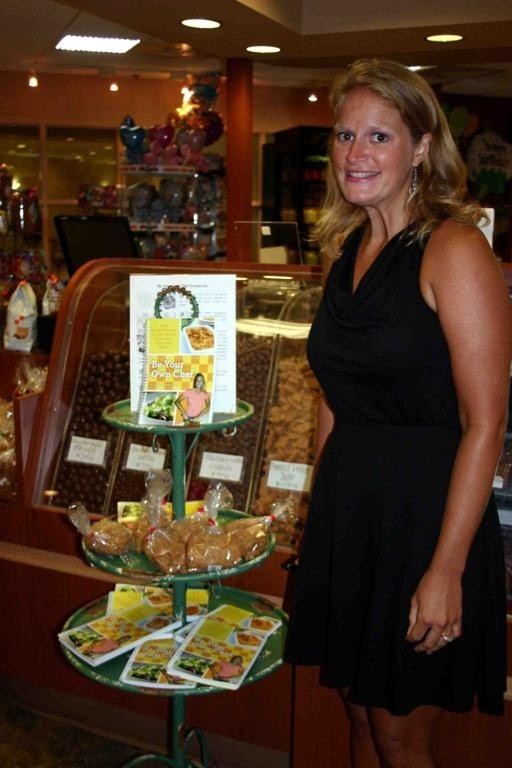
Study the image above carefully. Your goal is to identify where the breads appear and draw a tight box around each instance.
[87,510,270,574]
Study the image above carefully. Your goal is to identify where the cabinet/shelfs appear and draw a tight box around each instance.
[0,259,512,768]
[1,124,264,305]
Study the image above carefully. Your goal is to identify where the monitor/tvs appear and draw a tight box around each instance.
[53,214,140,278]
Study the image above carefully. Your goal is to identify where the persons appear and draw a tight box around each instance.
[83,635,132,657]
[210,656,244,683]
[287,58,512,768]
[175,373,210,426]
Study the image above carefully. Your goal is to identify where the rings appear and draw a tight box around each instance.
[441,635,453,642]
